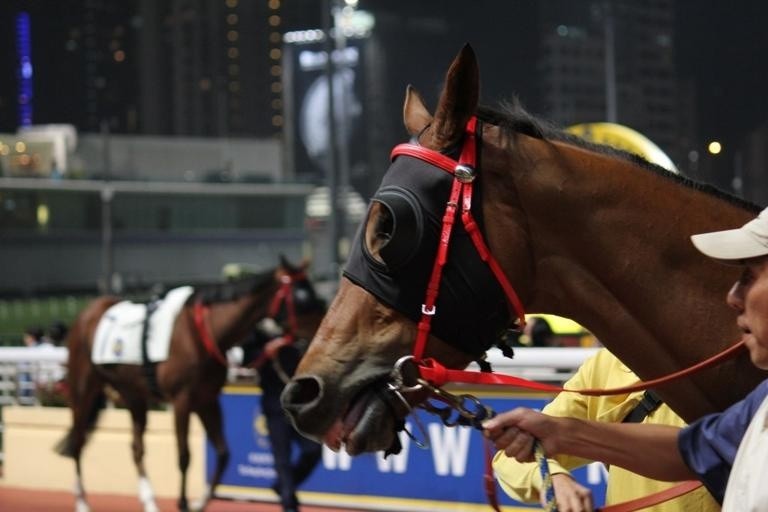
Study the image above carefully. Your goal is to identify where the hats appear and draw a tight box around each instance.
[687,206,768,268]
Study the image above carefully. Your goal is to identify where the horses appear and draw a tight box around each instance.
[279,41,768,457]
[51,253,327,512]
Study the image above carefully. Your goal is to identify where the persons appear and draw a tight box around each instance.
[235,335,325,511]
[485,346,723,512]
[11,320,73,409]
[478,201,767,512]
[521,315,552,347]
